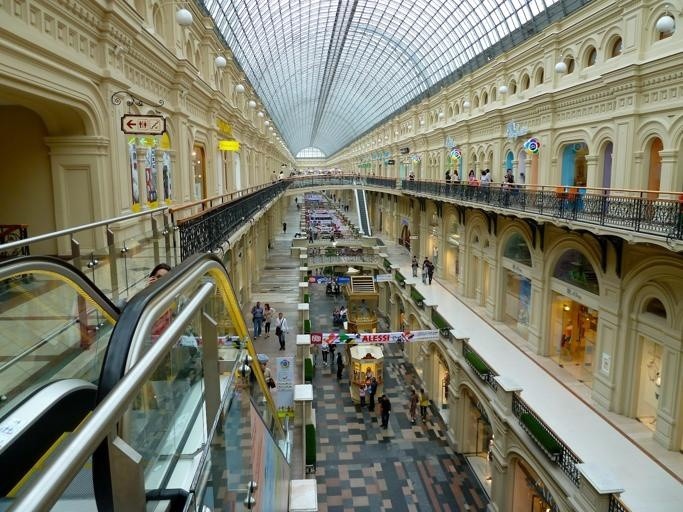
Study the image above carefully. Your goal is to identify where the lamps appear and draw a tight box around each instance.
[554,56,568,76]
[438,110,445,119]
[384,134,389,140]
[655,11,675,35]
[420,119,426,126]
[413,154,421,162]
[499,83,509,95]
[522,137,542,155]
[564,305,570,311]
[450,149,462,161]
[408,124,413,130]
[394,130,399,136]
[153,1,298,165]
[463,99,471,108]
[352,138,382,153]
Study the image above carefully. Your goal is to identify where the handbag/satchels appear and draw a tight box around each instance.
[276,327,283,336]
[270,378,276,388]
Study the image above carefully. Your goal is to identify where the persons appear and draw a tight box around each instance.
[270,171,284,184]
[446,170,491,202]
[412,256,435,285]
[410,389,428,425]
[359,377,391,428]
[333,305,348,328]
[145,264,203,413]
[262,363,271,401]
[337,352,345,378]
[275,313,289,350]
[251,302,276,339]
[326,283,340,295]
[321,344,336,366]
[283,223,286,232]
